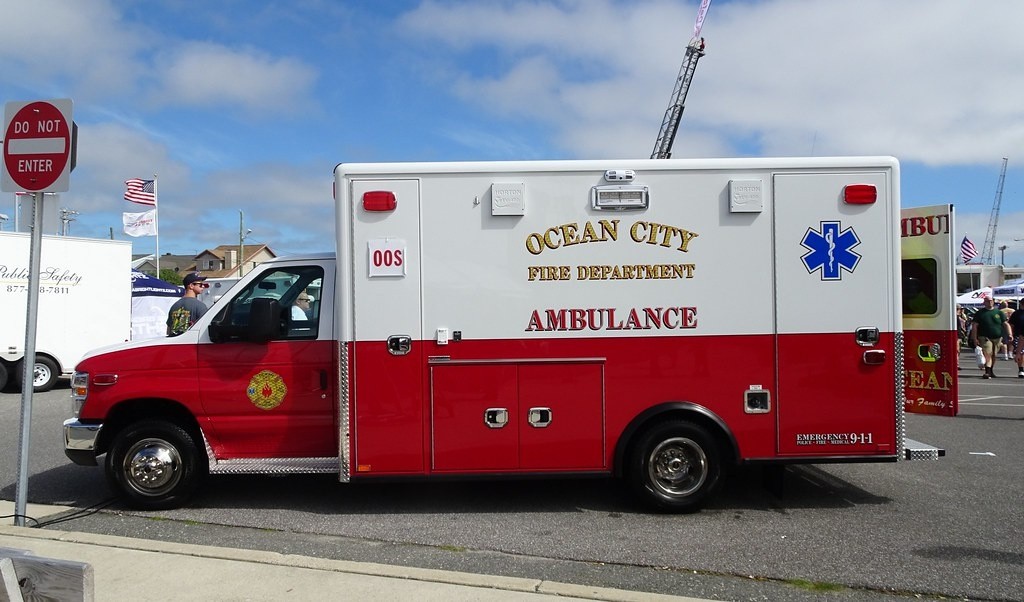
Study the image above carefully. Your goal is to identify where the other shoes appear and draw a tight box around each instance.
[1000,357,1008,360]
[1010,356,1012,358]
[1018,372,1024,379]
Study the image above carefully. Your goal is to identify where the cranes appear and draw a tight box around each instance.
[1013,238,1024,242]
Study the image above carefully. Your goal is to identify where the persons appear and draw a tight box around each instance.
[1015,324,1024,363]
[291,292,310,320]
[166,273,208,337]
[971,295,1014,378]
[957,299,1016,370]
[1008,298,1024,378]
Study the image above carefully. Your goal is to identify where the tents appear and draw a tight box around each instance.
[132,268,184,338]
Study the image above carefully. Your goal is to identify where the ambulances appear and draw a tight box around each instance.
[61,155,960,507]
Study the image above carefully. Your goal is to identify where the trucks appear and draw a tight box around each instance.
[198,276,322,320]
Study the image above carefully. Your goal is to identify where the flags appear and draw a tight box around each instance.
[961,237,979,262]
[123,178,155,205]
[123,209,156,238]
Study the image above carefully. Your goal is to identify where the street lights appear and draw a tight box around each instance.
[239,228,252,278]
[998,246,1006,264]
[980,157,1008,264]
[237,208,245,277]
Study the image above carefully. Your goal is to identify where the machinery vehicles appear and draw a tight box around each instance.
[645,36,706,159]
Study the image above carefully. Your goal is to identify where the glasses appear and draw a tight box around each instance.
[193,283,203,287]
[298,298,311,302]
[984,300,993,303]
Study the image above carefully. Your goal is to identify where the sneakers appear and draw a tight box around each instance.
[983,366,996,380]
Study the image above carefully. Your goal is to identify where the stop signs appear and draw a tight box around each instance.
[1,100,72,192]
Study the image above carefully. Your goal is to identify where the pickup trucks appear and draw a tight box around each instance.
[1,227,134,393]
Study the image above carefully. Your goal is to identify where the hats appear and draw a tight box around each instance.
[182,273,208,287]
